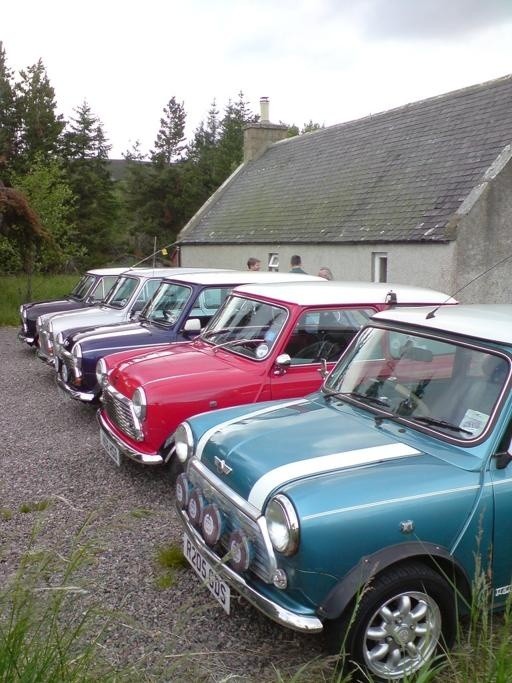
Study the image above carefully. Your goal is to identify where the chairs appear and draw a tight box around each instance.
[454,355,512,444]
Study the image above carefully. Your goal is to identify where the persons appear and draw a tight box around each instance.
[247,258,261,271]
[319,267,333,281]
[288,255,307,274]
